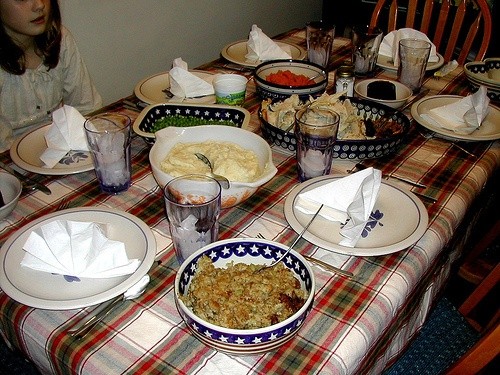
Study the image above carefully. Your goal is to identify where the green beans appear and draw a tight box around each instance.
[151,116,235,132]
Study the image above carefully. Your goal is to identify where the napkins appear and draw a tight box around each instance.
[20,220,142,277]
[293,167,383,248]
[168,58,215,102]
[40,104,99,169]
[378,28,439,62]
[245,24,292,64]
[420,85,490,136]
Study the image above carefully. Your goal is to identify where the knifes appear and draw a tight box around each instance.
[0,161,52,195]
[347,170,438,204]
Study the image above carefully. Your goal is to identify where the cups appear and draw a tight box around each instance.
[294,107,339,180]
[162,174,222,263]
[212,73,249,109]
[306,23,432,93]
[83,114,133,194]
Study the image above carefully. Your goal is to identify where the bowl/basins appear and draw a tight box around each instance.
[174,238,315,356]
[464,57,500,103]
[149,124,278,208]
[354,80,412,107]
[132,102,251,146]
[0,172,22,219]
[252,59,328,104]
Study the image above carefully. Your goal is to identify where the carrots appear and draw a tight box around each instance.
[265,70,317,86]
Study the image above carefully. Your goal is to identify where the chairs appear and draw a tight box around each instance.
[369,0,492,65]
[382,262,500,375]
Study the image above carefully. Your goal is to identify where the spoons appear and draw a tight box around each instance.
[354,163,427,188]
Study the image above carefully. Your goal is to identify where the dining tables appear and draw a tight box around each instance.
[0,26,500,375]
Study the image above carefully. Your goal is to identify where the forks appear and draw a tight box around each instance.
[417,127,478,160]
[255,234,353,279]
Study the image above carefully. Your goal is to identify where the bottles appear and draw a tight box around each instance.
[334,60,356,98]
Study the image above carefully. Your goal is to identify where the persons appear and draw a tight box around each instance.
[0,0,104,157]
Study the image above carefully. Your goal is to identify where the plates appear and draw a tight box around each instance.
[222,38,306,67]
[135,69,216,104]
[10,115,125,175]
[376,51,444,72]
[284,175,428,258]
[0,205,156,310]
[411,94,500,142]
